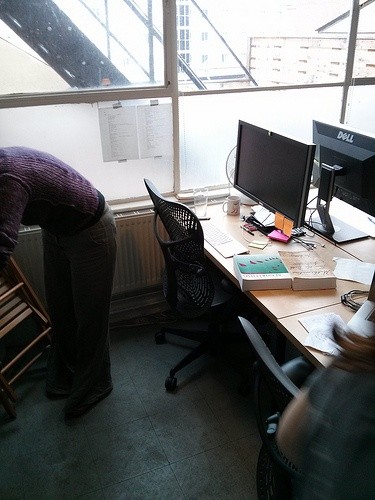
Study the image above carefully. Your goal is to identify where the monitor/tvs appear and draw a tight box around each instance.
[234,119,316,237]
[304,119,375,243]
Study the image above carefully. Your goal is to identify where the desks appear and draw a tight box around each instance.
[166,185,374,372]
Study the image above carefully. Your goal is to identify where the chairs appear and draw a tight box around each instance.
[0,255,51,417]
[237,316,301,500]
[143,179,247,392]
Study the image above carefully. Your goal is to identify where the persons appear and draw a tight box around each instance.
[276,325,375,500]
[0,146,118,420]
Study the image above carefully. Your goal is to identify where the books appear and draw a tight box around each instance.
[278,250,337,290]
[233,252,292,292]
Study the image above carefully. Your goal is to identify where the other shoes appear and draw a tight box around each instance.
[45,388,68,400]
[62,387,112,418]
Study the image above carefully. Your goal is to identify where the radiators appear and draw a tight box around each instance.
[12,207,169,311]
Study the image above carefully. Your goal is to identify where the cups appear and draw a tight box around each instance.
[222,195,240,215]
[193,187,209,217]
